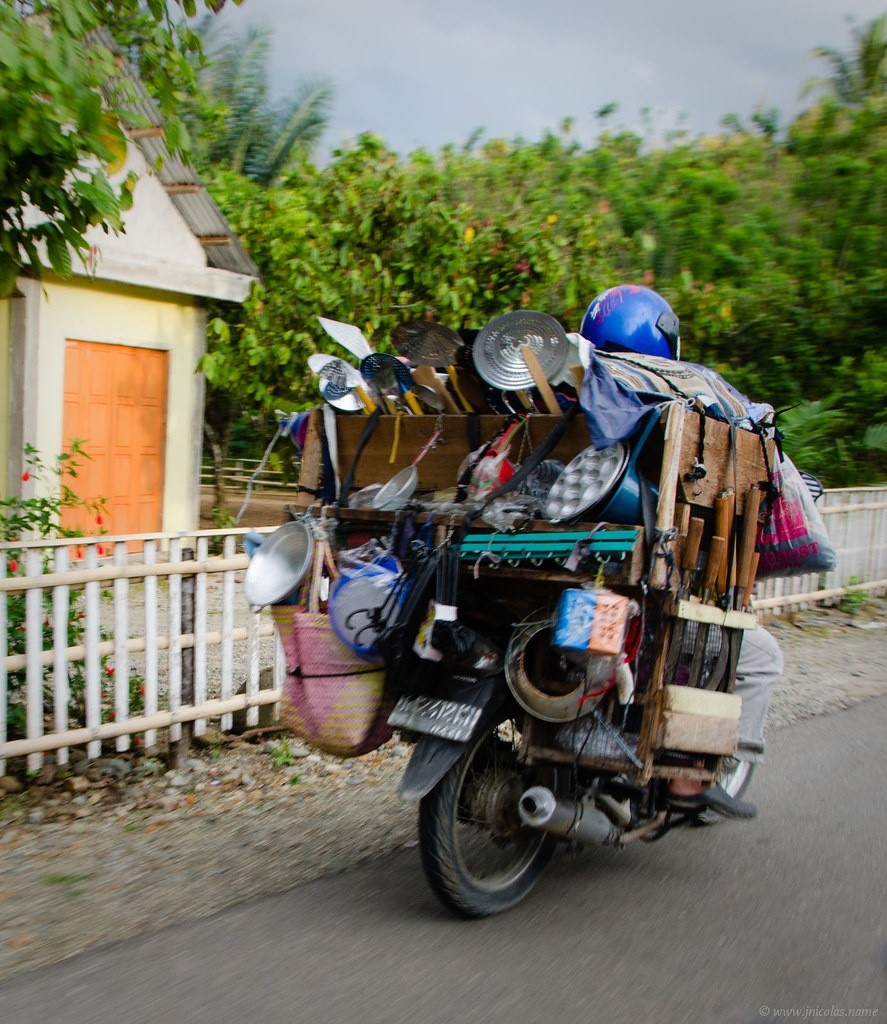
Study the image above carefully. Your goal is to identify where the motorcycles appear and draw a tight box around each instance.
[387,585,760,921]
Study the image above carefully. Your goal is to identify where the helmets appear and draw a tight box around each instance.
[579,284,679,361]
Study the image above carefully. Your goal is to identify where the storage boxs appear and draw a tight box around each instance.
[312,402,774,522]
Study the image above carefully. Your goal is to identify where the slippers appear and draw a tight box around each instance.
[656,779,757,820]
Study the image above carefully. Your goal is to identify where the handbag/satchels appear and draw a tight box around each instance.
[754,441,836,581]
[271,538,398,759]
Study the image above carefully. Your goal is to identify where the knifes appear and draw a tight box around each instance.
[665,484,762,694]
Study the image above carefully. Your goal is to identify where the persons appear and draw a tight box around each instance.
[578,282,785,822]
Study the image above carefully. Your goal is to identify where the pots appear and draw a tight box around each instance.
[244,520,312,614]
